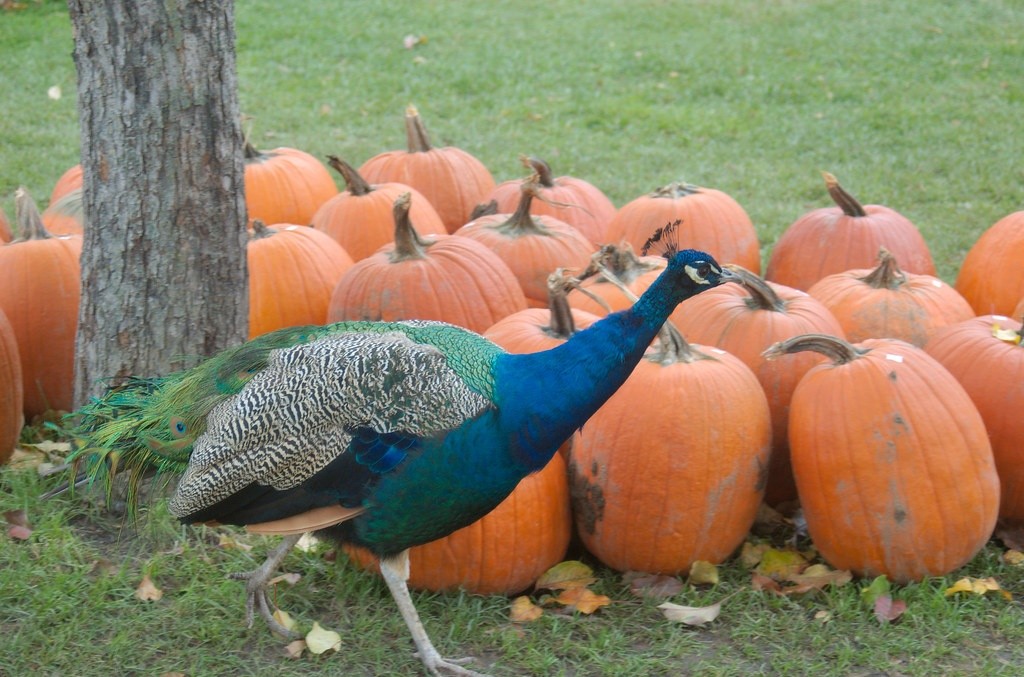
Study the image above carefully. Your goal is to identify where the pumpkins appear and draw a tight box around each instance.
[242,105,1024,594]
[0,163,84,467]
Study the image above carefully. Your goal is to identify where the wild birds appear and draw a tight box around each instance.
[65,221,752,677]
